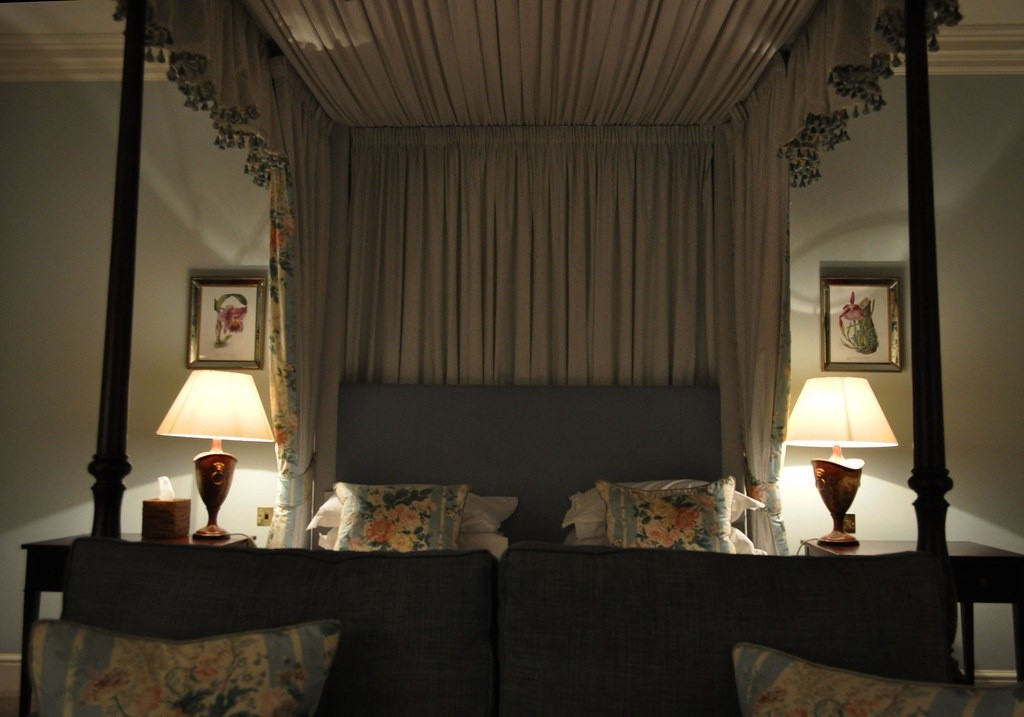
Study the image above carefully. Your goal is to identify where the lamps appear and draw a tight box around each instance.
[784,377,900,548]
[156,370,276,540]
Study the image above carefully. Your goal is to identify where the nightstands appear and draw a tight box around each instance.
[801,538,1024,687]
[19,533,256,716]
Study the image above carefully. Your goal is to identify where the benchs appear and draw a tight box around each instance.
[63,537,954,717]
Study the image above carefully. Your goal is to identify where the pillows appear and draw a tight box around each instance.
[733,641,1024,717]
[26,614,347,717]
[561,475,769,556]
[304,480,518,561]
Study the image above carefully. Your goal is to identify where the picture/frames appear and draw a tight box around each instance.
[818,277,902,373]
[185,276,267,369]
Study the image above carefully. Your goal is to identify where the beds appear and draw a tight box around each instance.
[87,0,962,554]
